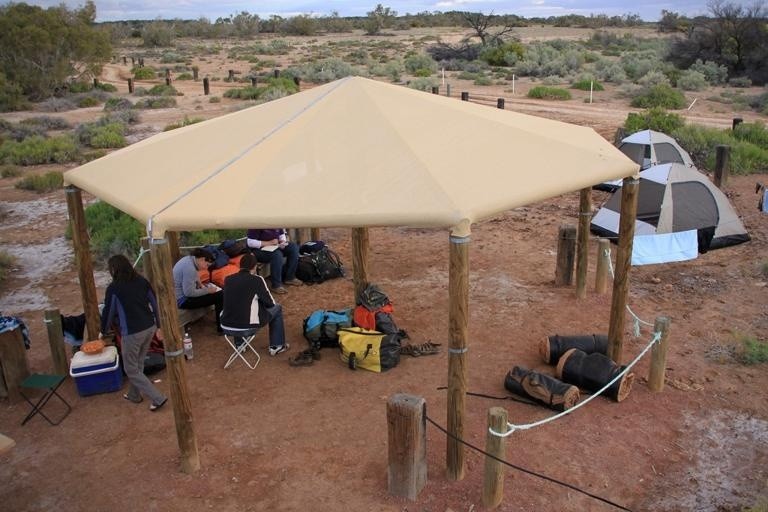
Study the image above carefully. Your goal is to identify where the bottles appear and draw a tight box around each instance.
[183,333,194,360]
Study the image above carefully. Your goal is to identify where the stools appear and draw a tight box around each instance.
[17,370,73,427]
[219,319,263,371]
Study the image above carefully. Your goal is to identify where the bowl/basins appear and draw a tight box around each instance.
[80,339,105,354]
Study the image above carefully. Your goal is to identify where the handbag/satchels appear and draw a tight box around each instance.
[302,308,351,349]
[336,327,402,373]
[352,285,405,337]
[296,240,344,282]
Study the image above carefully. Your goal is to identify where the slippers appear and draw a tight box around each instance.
[124,392,143,402]
[149,398,168,411]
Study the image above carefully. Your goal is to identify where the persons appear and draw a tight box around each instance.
[173,249,224,335]
[220,254,290,356]
[97,255,167,411]
[164,68,174,85]
[247,226,302,294]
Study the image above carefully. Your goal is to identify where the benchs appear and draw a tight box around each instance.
[177,253,289,343]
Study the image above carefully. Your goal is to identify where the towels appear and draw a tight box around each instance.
[630,228,699,266]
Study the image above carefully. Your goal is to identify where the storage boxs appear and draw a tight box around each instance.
[69,348,125,395]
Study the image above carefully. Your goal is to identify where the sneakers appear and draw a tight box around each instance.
[400,339,442,356]
[289,348,321,366]
[285,278,303,286]
[269,342,290,356]
[271,286,288,294]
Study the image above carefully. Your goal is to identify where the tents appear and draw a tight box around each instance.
[591,129,697,193]
[591,161,753,252]
